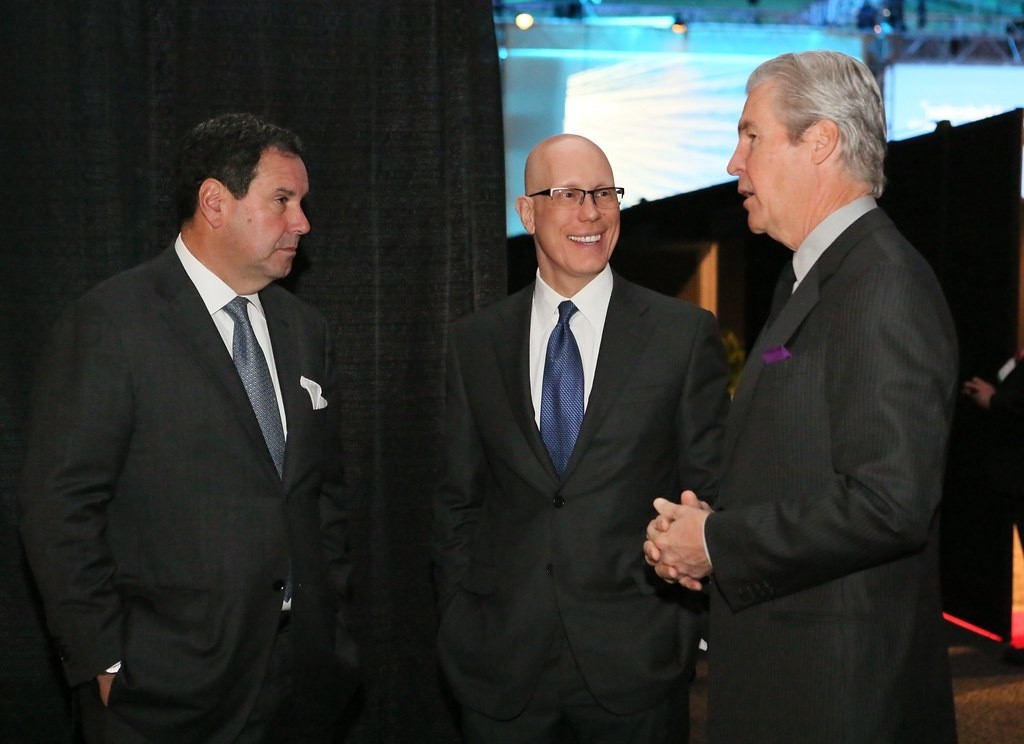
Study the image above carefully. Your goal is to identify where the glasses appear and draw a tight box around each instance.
[527,187,625,209]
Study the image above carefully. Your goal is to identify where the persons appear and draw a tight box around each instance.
[12,112,345,744]
[960,340,1024,554]
[428,133,732,744]
[642,51,960,744]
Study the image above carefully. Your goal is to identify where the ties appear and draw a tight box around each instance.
[540,301,584,479]
[223,294,291,603]
[766,259,795,333]
